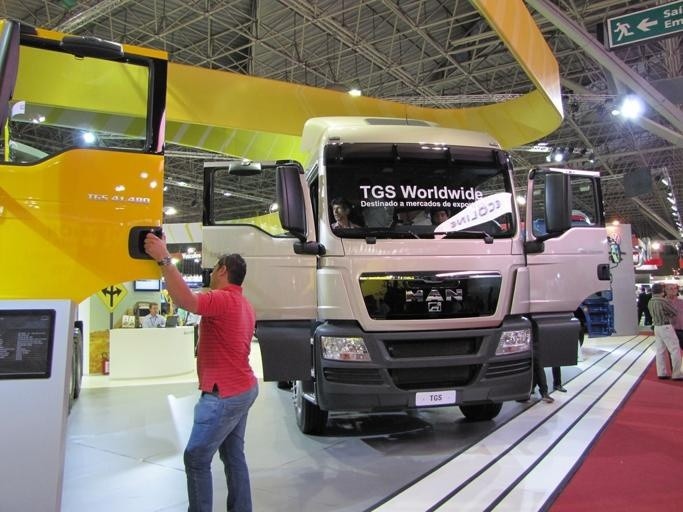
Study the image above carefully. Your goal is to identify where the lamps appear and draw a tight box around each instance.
[660,167,682,243]
[545,147,596,165]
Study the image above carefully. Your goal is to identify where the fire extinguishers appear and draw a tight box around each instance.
[102,358,110,375]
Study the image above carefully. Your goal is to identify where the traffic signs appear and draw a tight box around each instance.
[607,1,683,50]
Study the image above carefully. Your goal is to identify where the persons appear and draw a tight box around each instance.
[141,302,167,328]
[331,197,361,230]
[141,232,259,511]
[635,283,682,381]
[184,310,202,357]
[529,364,554,402]
[551,366,568,393]
[431,209,450,233]
[611,20,636,42]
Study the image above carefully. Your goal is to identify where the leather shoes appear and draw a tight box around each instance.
[554,384,567,392]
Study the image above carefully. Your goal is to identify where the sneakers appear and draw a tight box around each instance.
[542,394,553,402]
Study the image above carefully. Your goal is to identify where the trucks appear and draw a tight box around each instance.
[199,114,616,442]
[521,214,617,352]
[0,13,171,423]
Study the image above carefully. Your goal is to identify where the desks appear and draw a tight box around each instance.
[108,324,196,380]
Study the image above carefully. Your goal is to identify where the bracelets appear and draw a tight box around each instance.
[155,255,173,267]
